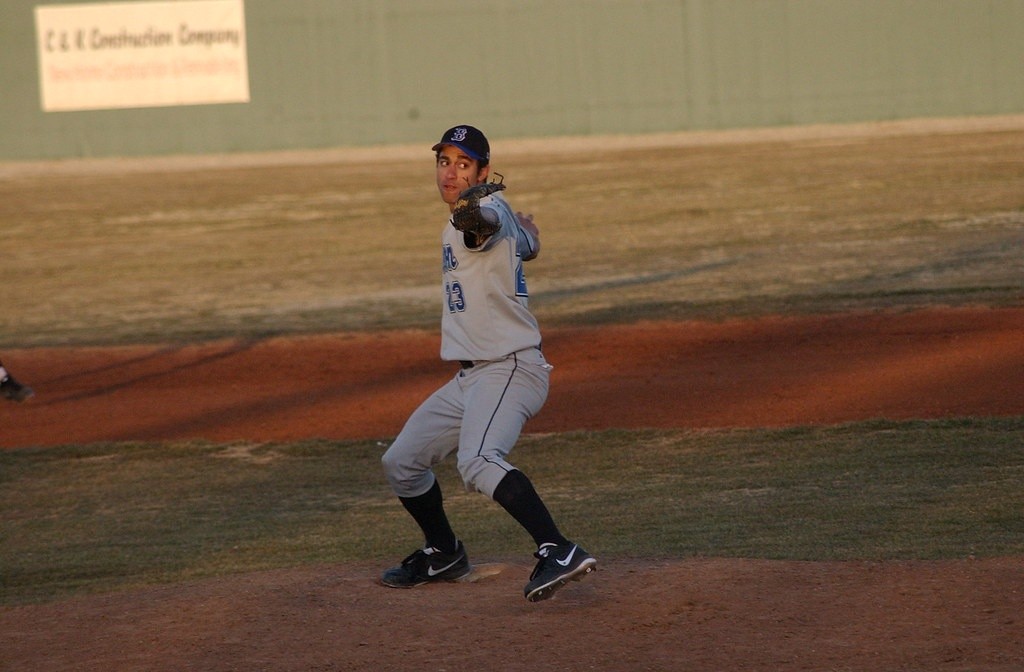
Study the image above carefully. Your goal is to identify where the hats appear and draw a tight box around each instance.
[432,125,490,165]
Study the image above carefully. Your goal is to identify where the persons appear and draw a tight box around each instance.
[380,124,597,602]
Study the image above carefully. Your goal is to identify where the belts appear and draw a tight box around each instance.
[460,343,541,370]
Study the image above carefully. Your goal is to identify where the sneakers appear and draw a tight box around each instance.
[380,540,470,589]
[0,375,35,404]
[525,542,597,602]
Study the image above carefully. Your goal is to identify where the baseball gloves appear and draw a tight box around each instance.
[449,171,506,246]
